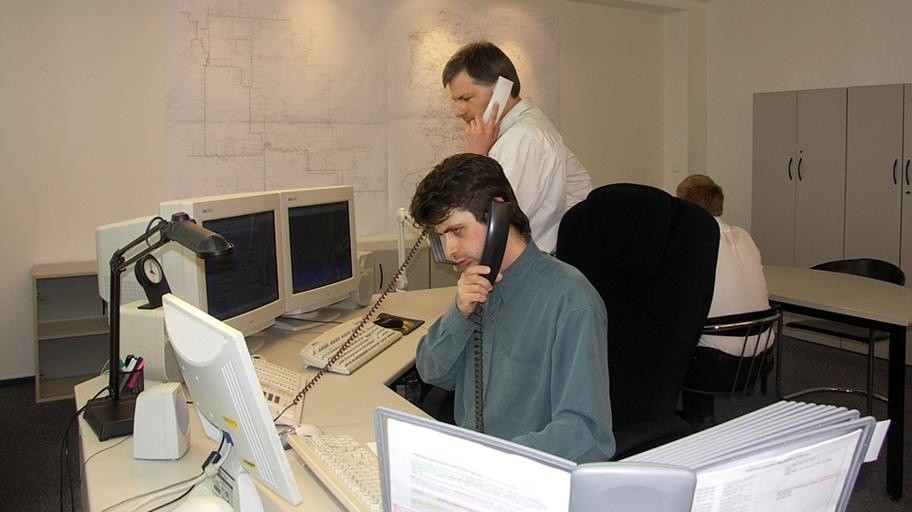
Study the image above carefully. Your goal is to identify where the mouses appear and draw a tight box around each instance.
[277,422,324,450]
[375,314,405,328]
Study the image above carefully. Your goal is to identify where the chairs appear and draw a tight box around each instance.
[680,307,781,417]
[773,253,907,426]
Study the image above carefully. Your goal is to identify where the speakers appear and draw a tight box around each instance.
[130,382,193,463]
[119,297,187,382]
[350,251,375,307]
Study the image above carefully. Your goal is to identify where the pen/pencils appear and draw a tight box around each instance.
[117,355,145,396]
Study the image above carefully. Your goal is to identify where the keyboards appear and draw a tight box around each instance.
[287,431,382,512]
[300,315,403,375]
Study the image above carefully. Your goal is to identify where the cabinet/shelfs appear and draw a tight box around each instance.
[839,84,911,278]
[28,260,113,405]
[750,82,848,267]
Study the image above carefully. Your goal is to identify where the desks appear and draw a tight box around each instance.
[74,258,911,512]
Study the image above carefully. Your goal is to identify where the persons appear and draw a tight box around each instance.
[441,41,594,259]
[411,152,619,465]
[677,175,774,427]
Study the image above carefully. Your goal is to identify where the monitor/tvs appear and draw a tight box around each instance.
[160,292,304,512]
[281,185,361,331]
[94,191,286,354]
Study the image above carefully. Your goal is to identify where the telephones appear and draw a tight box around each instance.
[479,199,513,286]
[482,75,514,127]
